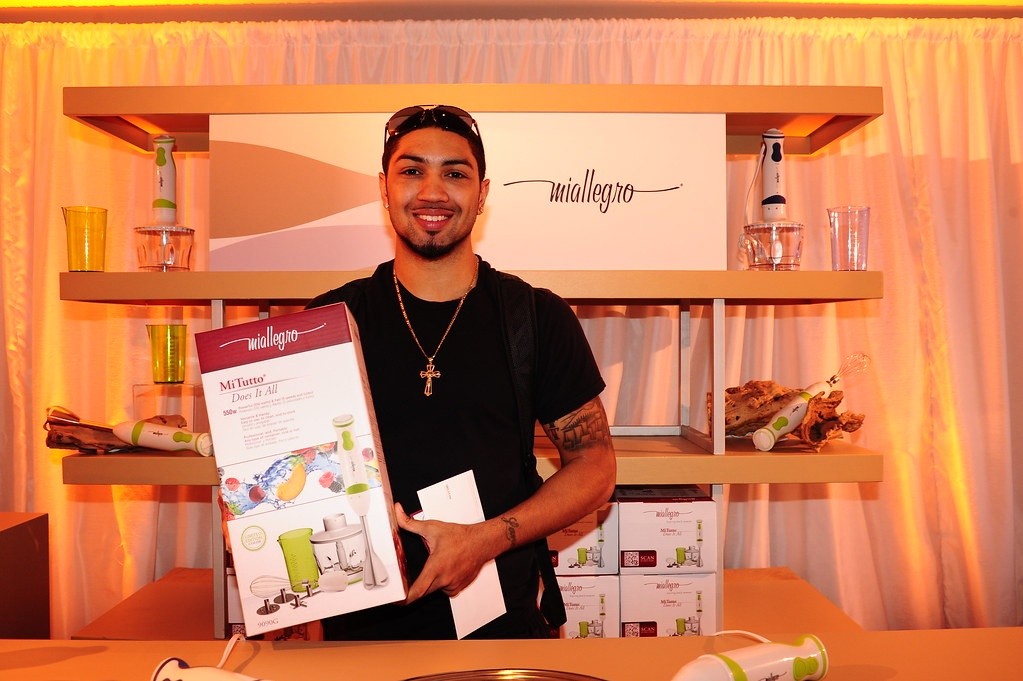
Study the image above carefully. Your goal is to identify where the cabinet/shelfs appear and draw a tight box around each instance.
[57,84,885,641]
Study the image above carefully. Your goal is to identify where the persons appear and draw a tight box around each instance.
[217,104,617,640]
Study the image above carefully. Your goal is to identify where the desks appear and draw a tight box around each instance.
[0,626,1023,681]
[0,510,51,643]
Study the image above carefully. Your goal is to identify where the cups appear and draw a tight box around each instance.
[676,618,685,634]
[676,547,685,563]
[826,205,871,271]
[579,621,589,638]
[145,324,188,383]
[577,548,587,564]
[277,528,320,593]
[62,206,108,272]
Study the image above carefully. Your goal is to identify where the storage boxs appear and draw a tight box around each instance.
[194,302,407,642]
[534,486,720,636]
[207,114,728,271]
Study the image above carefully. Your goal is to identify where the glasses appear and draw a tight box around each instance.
[384,105,482,150]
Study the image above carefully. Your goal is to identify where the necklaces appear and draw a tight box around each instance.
[393,254,479,395]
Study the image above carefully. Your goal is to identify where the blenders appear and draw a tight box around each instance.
[133,134,196,272]
[743,129,805,271]
